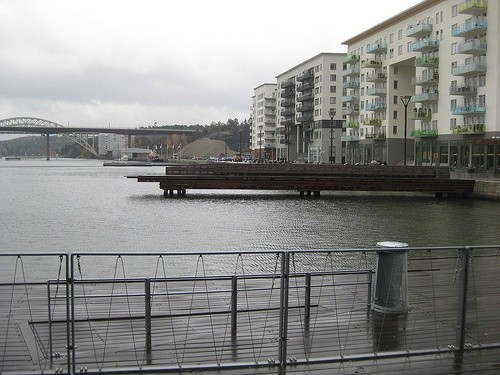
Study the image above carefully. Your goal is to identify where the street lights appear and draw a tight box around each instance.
[286,119,292,162]
[238,129,243,158]
[258,125,263,158]
[400,94,412,166]
[328,108,337,165]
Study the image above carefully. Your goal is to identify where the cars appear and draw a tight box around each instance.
[169,153,389,168]
